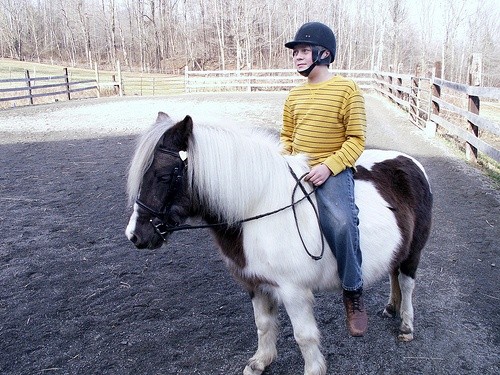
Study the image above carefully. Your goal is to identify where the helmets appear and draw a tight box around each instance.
[284,22,336,63]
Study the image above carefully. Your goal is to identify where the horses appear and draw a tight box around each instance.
[123,112,433,375]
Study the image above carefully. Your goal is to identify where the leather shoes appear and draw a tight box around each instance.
[343,289,368,337]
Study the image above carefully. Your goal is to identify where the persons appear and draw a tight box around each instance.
[281,23,369,336]
